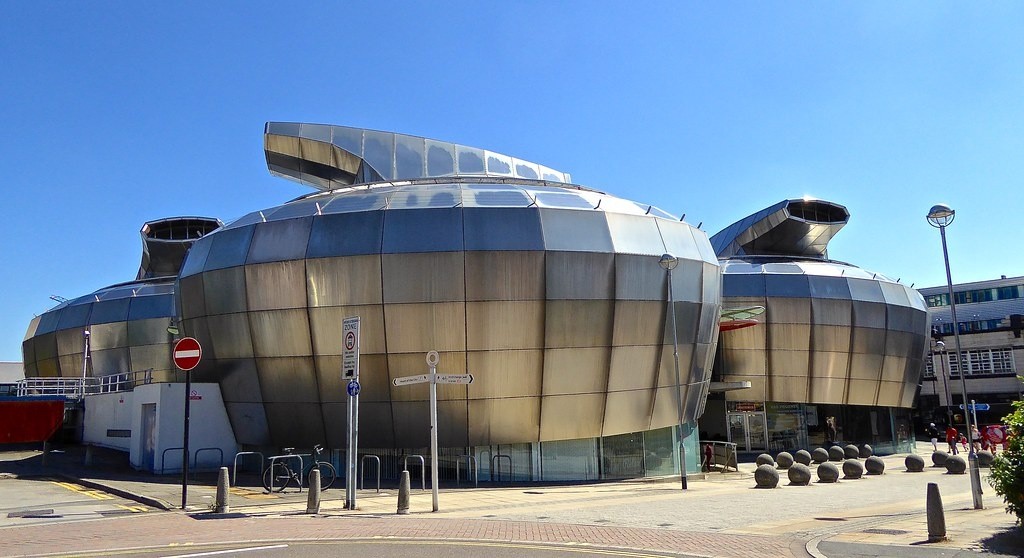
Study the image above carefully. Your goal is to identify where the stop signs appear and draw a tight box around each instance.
[172,336,203,370]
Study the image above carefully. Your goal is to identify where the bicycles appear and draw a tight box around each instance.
[262,442,337,493]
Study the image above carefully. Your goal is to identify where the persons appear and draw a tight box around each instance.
[704,444,712,472]
[958,433,968,452]
[929,423,939,451]
[971,425,980,453]
[945,424,959,454]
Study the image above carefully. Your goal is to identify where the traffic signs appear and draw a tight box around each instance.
[392,374,430,387]
[435,373,475,385]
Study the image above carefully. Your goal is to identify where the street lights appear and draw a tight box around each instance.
[935,340,955,429]
[656,254,690,492]
[925,202,985,511]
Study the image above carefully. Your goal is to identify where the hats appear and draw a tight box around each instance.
[930,423,935,427]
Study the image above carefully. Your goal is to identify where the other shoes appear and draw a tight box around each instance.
[947,451,951,453]
